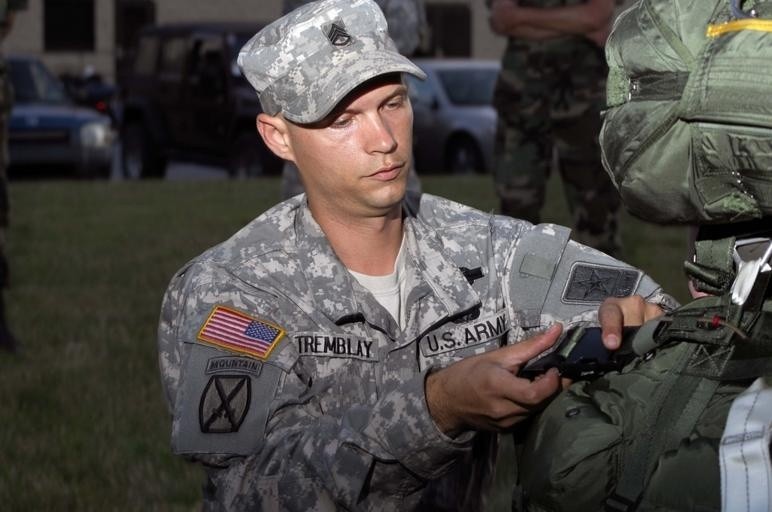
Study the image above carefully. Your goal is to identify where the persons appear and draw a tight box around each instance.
[0,0,30,354]
[483,0,623,256]
[84,68,120,130]
[158,1,683,512]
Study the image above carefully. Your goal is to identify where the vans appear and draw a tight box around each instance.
[118,25,287,180]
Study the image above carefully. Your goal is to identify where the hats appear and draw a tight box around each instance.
[236,0,426,124]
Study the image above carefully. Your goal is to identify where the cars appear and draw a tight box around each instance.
[0,59,122,180]
[405,59,500,174]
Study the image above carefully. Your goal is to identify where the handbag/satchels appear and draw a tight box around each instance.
[458,296,771,511]
[598,0,772,223]
